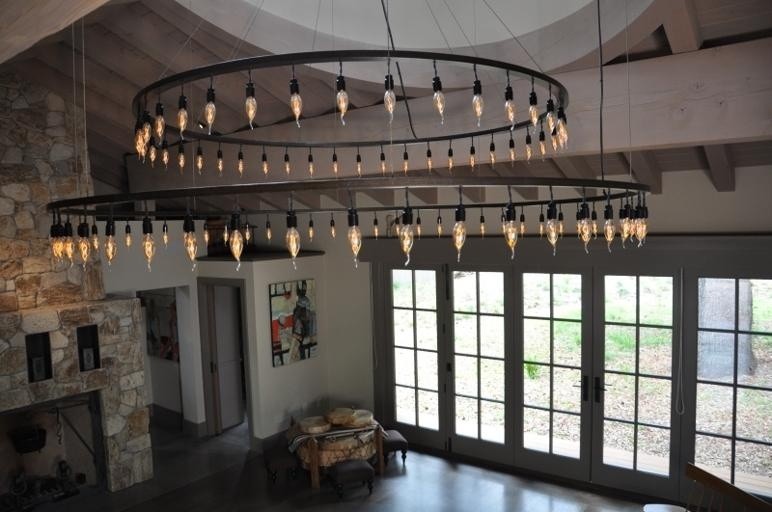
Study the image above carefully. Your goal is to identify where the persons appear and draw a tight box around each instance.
[292,280,311,361]
[282,289,292,316]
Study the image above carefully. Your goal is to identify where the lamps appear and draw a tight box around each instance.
[47,0,651,271]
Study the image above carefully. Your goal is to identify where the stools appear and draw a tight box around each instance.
[327,458,376,499]
[263,442,309,501]
[382,430,408,466]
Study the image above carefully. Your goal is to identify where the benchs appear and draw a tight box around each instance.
[642,460,772,512]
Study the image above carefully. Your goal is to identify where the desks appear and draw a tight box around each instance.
[279,419,388,489]
